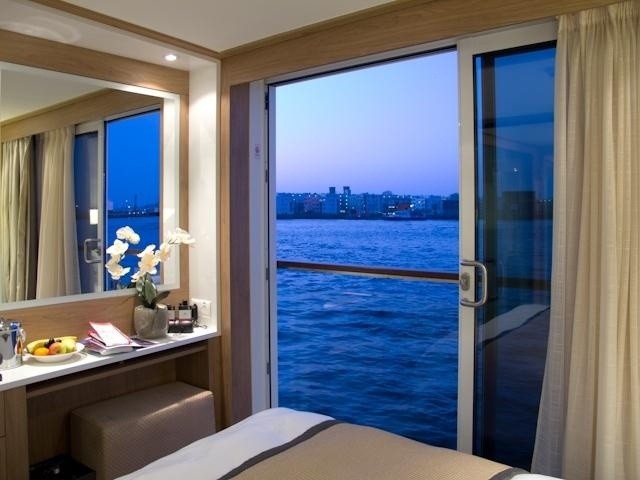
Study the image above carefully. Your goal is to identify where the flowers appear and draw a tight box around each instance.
[130,226,196,310]
[103,225,140,290]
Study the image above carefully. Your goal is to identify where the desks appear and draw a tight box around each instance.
[0,325,224,480]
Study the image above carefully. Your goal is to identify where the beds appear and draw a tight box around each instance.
[110,404,565,480]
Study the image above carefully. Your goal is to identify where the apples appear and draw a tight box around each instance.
[49,343,67,355]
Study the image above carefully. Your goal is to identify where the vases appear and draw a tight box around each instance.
[134,304,169,340]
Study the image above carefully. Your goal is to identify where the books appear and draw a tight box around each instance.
[85,321,157,357]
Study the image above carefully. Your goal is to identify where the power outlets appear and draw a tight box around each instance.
[191,299,211,320]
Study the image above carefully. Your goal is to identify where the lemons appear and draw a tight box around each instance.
[34,347,49,356]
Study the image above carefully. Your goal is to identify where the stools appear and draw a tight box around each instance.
[67,380,218,480]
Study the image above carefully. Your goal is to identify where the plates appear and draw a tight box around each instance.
[24,343,85,363]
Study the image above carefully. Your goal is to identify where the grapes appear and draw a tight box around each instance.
[44,338,61,348]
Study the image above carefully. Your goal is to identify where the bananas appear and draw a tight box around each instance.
[26,335,78,353]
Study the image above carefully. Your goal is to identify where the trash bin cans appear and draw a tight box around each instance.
[29,453,97,480]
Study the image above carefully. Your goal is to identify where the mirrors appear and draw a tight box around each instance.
[0,29,190,345]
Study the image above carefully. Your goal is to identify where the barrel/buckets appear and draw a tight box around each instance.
[0,317,26,371]
